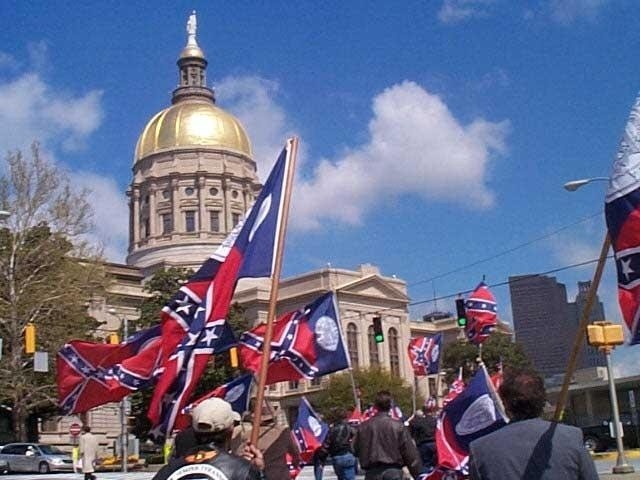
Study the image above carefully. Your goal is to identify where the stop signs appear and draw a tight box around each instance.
[69,423,81,437]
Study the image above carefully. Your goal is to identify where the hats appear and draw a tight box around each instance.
[192,396,241,434]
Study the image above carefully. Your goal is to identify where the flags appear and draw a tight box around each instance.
[407,331,441,376]
[56,318,238,415]
[285,396,328,479]
[423,369,509,478]
[145,139,292,444]
[236,292,349,386]
[464,281,498,345]
[604,90,640,346]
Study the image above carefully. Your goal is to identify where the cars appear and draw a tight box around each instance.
[0,442,74,474]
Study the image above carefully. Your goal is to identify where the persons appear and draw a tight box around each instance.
[468,365,600,478]
[311,390,437,480]
[151,396,258,478]
[77,426,99,480]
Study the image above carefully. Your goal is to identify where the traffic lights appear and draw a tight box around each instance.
[372,316,385,344]
[454,298,468,326]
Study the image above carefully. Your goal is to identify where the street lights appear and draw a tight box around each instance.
[564,177,610,192]
